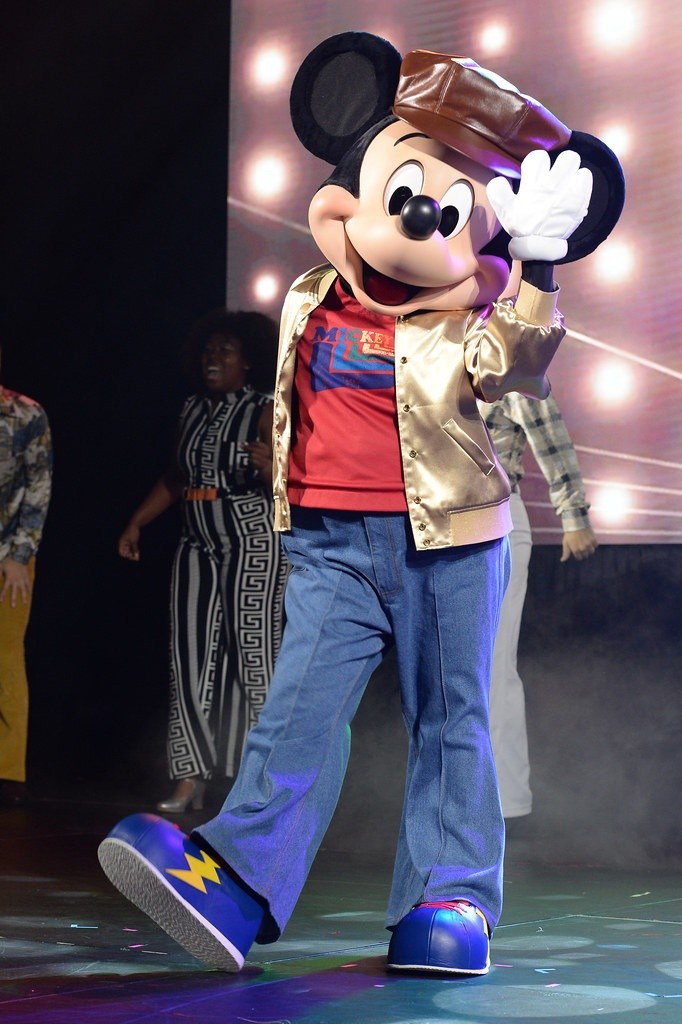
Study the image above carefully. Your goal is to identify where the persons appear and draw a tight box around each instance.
[472,383,599,820]
[0,384,53,809]
[122,310,293,815]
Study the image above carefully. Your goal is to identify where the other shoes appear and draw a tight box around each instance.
[5,781,29,805]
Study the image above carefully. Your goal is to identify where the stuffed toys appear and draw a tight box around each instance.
[97,32,625,974]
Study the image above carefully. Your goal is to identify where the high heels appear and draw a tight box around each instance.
[157,777,204,812]
